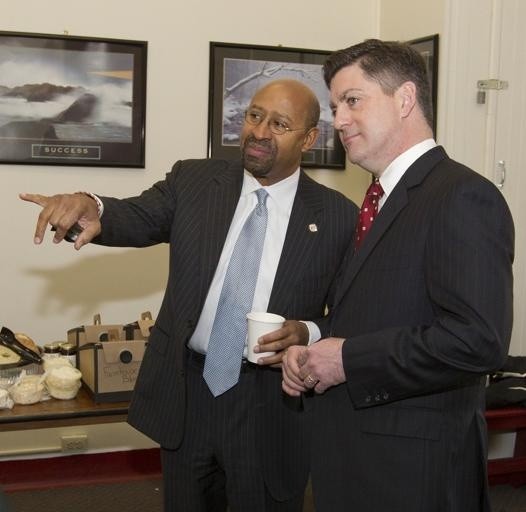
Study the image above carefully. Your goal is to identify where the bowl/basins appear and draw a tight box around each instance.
[9,368,82,405]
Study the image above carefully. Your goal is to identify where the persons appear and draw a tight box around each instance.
[18,80,363,511]
[280,37,516,512]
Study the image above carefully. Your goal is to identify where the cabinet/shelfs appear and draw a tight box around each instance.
[0,361,524,494]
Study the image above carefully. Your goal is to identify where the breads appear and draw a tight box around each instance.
[15,333,40,355]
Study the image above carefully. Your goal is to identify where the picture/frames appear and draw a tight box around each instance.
[1,28,145,170]
[205,33,440,170]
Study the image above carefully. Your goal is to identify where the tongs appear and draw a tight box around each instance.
[0,326,45,364]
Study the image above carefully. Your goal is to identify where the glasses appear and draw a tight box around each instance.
[245,110,308,135]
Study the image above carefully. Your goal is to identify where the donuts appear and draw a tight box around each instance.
[0,344,21,364]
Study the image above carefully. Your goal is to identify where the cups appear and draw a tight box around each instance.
[245,313,285,364]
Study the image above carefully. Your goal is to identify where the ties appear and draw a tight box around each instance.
[203,188,268,398]
[354,184,384,255]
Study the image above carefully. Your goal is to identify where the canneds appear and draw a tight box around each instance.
[44,342,77,368]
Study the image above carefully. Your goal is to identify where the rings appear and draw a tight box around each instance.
[306,374,316,385]
